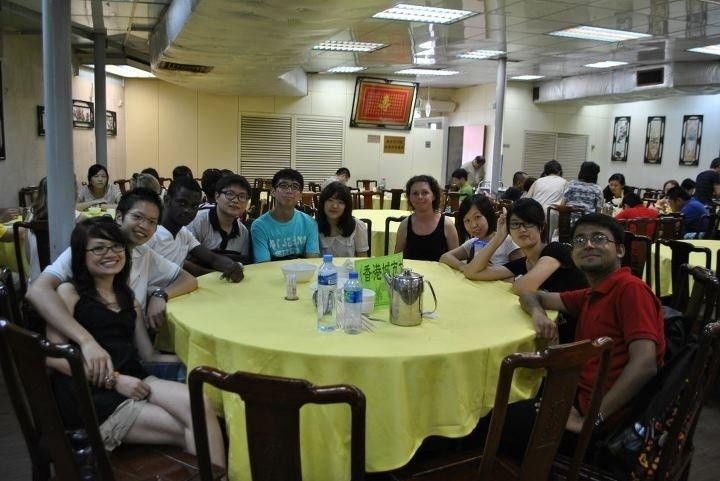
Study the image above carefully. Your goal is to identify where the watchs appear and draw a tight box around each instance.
[595,410,605,429]
[102,371,121,390]
[151,288,169,303]
[233,262,244,271]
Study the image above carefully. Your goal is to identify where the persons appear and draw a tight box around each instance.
[445,169,472,216]
[465,198,587,342]
[652,180,681,213]
[180,175,249,276]
[561,162,605,227]
[528,161,569,229]
[666,187,712,240]
[173,165,193,178]
[438,194,527,273]
[27,176,92,289]
[613,193,658,258]
[74,164,123,210]
[24,187,200,399]
[394,174,459,261]
[601,173,634,204]
[520,177,537,199]
[682,179,696,197]
[251,169,319,263]
[321,167,351,191]
[478,212,665,457]
[316,181,371,256]
[461,155,486,194]
[142,176,244,283]
[131,174,161,195]
[0,207,30,242]
[202,169,225,204]
[42,217,228,480]
[694,157,719,204]
[502,172,530,201]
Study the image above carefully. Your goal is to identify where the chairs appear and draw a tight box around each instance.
[671,262,719,345]
[353,190,383,211]
[253,178,273,191]
[114,180,131,195]
[654,216,684,238]
[308,182,322,194]
[0,322,226,481]
[189,366,367,481]
[618,216,659,239]
[654,236,712,313]
[241,188,271,230]
[414,338,612,479]
[384,216,409,258]
[442,192,469,219]
[381,189,408,210]
[621,230,652,288]
[13,220,51,296]
[559,319,719,481]
[159,177,172,192]
[546,204,587,246]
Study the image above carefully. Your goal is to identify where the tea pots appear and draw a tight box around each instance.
[382,267,438,328]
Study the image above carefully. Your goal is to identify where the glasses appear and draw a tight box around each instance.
[85,242,125,255]
[571,234,621,245]
[509,220,537,229]
[221,191,249,202]
[275,182,300,190]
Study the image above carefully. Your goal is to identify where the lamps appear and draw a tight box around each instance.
[425,86,431,117]
[81,58,157,77]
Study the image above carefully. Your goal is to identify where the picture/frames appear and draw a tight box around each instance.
[350,76,419,131]
[644,116,666,163]
[105,110,117,135]
[38,105,45,135]
[72,99,94,128]
[611,116,631,161]
[678,114,703,166]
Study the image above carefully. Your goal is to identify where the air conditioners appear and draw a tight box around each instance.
[421,100,456,112]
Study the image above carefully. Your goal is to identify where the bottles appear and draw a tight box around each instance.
[604,202,612,217]
[344,272,362,335]
[317,255,338,332]
[381,178,386,187]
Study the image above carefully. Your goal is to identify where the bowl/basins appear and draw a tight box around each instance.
[363,301,374,312]
[362,289,376,301]
[280,263,316,283]
